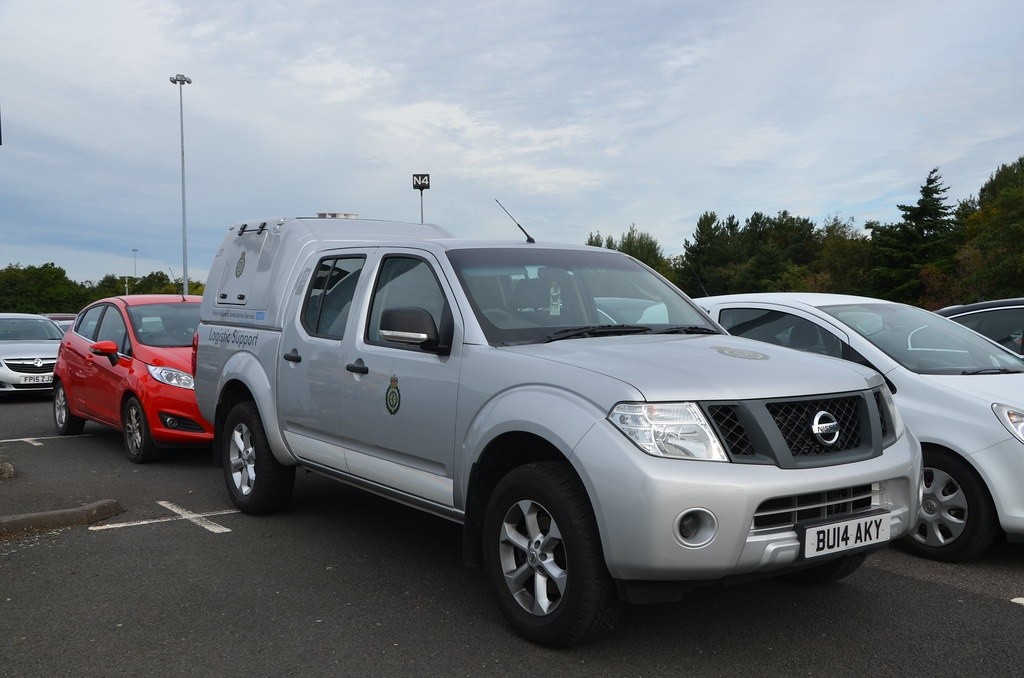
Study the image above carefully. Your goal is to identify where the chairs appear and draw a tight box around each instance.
[746,316,784,346]
[109,309,199,354]
[462,270,485,311]
[507,278,558,327]
[788,321,821,355]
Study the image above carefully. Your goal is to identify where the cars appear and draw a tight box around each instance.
[0,313,65,394]
[691,291,1024,566]
[51,294,220,462]
[930,298,1024,358]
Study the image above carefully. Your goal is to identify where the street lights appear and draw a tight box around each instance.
[170,74,192,295]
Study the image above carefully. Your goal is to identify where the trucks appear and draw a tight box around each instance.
[190,211,924,651]
